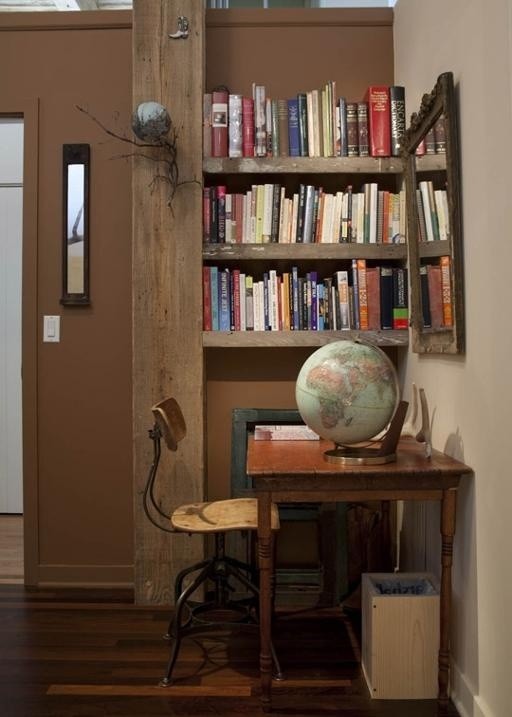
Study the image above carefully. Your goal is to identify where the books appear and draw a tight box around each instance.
[253,423,320,441]
[203,183,407,244]
[409,116,455,329]
[202,81,407,158]
[202,259,407,331]
[271,565,326,613]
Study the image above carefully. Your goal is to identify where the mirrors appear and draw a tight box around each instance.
[60,143,91,306]
[397,70,467,355]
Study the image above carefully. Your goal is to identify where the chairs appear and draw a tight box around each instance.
[143,394,289,684]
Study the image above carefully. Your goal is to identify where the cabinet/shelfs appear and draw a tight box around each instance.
[202,156,409,348]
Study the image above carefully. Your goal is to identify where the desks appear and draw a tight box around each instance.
[245,425,473,707]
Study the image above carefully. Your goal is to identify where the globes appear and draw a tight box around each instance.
[294,334,409,465]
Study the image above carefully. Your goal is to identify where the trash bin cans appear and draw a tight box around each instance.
[361,572,440,699]
